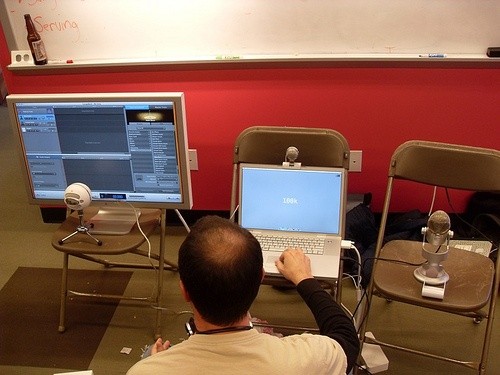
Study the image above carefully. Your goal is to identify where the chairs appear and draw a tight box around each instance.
[224,122,350,346]
[50,201,181,344]
[352,138,500,373]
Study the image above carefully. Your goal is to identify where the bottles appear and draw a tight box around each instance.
[23,14,48,65]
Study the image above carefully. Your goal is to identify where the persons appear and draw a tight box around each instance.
[124,215,362,375]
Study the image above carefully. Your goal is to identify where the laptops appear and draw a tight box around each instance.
[239,163,345,281]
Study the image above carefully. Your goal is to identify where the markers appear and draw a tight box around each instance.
[47,60,73,65]
[418,54,445,58]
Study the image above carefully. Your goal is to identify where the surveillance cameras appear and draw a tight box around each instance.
[57,183,102,246]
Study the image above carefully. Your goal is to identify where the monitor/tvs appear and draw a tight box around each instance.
[5,91,193,210]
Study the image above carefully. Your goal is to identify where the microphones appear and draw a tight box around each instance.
[421,210,454,246]
[285,146,299,162]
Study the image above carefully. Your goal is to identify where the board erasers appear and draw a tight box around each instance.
[487,47,500,57]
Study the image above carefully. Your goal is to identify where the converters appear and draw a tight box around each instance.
[357,331,390,374]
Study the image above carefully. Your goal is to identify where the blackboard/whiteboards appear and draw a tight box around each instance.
[0,1,500,67]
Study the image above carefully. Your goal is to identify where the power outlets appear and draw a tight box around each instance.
[347,149,362,172]
[186,148,199,171]
